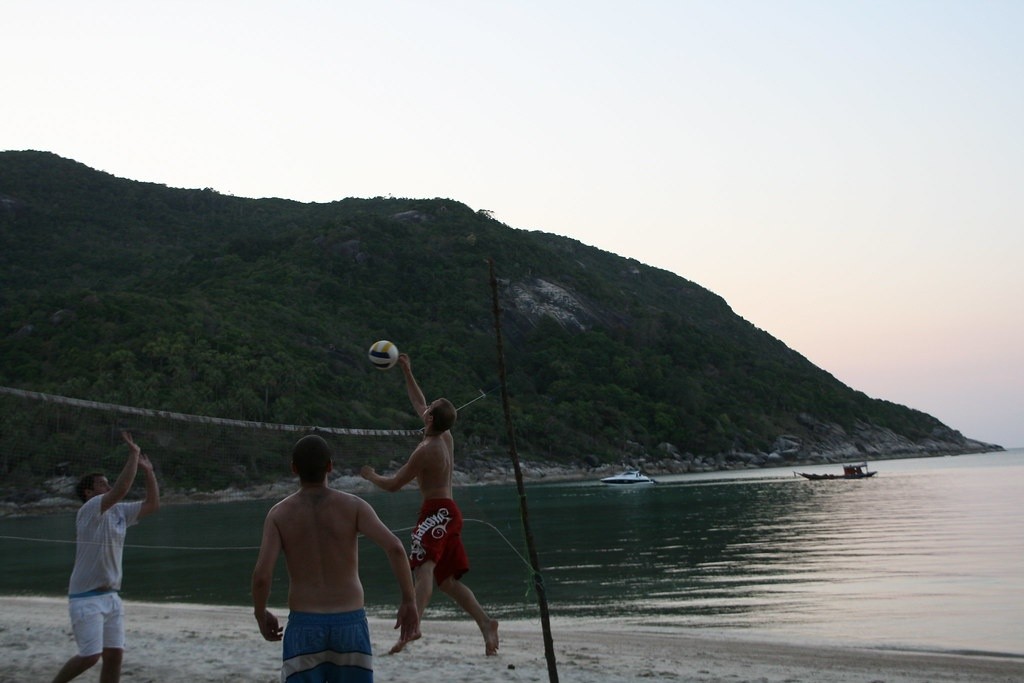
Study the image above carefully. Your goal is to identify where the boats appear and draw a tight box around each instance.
[794,461,878,481]
[600,470,657,485]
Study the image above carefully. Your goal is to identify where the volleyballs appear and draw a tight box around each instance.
[369,339,398,370]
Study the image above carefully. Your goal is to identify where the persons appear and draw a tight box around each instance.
[360,353,500,655]
[249,435,421,682]
[51,431,161,683]
